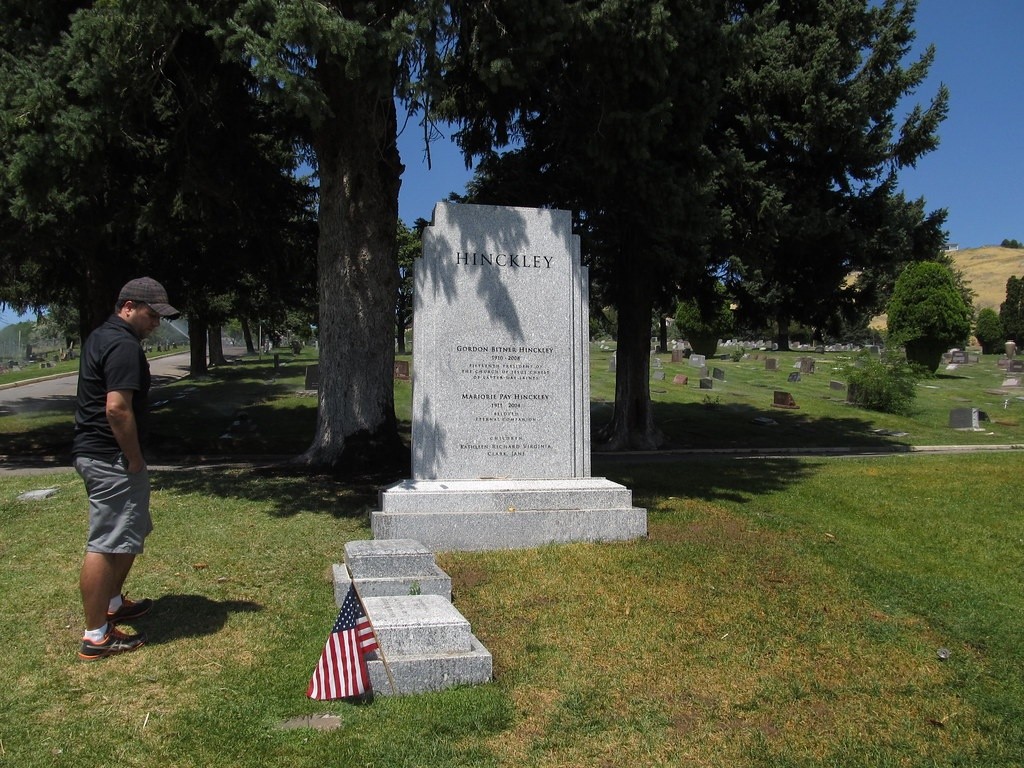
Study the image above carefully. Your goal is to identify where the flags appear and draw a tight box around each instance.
[308,584,379,701]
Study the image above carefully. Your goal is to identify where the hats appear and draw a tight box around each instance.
[118,276,181,320]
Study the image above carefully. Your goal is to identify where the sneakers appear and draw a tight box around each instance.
[107,591,154,623]
[78,623,148,659]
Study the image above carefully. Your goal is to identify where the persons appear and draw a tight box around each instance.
[74,277,178,659]
